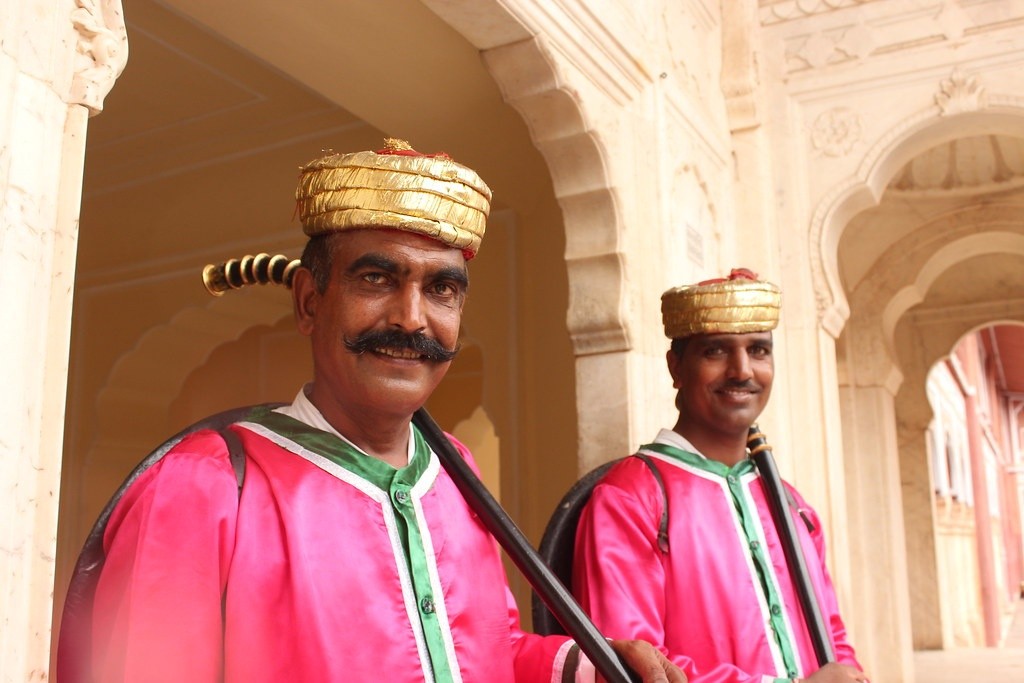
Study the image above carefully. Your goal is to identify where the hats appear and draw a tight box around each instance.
[661,268,781,338]
[298,138,493,260]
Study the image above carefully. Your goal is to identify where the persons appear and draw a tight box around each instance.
[61,137,696,682]
[574,270,871,683]
